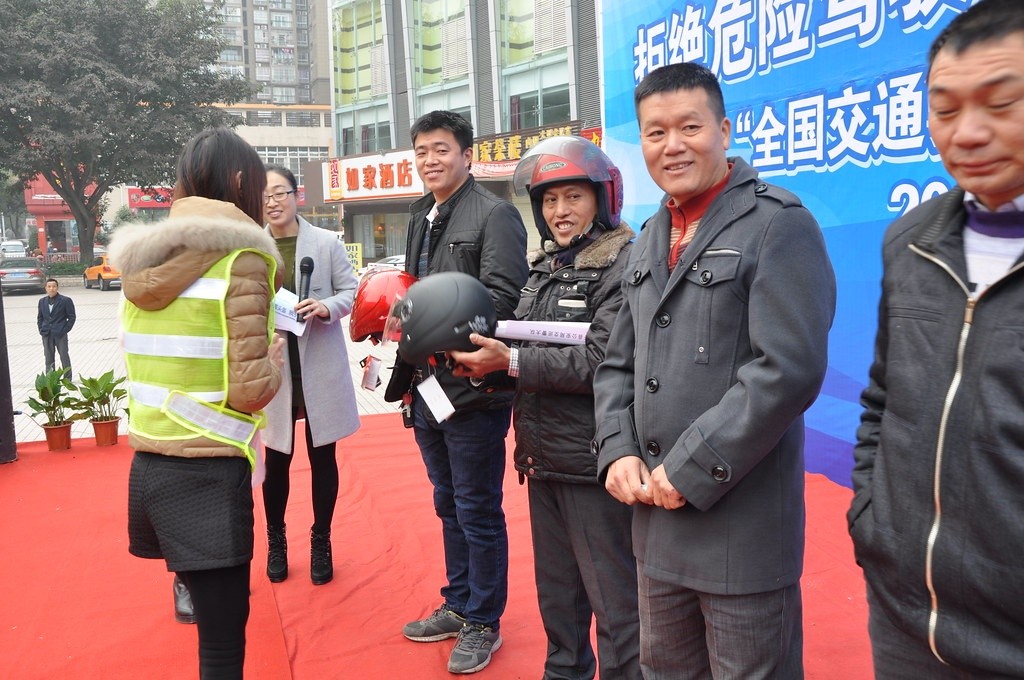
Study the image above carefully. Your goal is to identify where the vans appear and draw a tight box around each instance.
[0,240,29,259]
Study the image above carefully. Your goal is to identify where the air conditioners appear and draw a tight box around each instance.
[261,25,267,30]
[302,11,307,15]
[262,82,266,85]
[302,30,307,34]
[261,44,266,48]
[255,25,260,29]
[262,101,268,104]
[304,85,310,89]
[309,5,312,9]
[259,7,264,10]
[254,44,260,48]
[256,63,261,67]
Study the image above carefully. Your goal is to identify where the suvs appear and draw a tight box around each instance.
[83,255,123,291]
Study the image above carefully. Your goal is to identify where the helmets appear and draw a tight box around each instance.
[528,137,623,239]
[393,269,497,367]
[350,266,418,341]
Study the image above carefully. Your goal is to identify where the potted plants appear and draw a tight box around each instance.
[21,361,130,451]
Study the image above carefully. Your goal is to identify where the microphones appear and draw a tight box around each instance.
[296,257,314,323]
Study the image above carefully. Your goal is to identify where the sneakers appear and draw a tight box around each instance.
[402,603,466,642]
[447,620,503,673]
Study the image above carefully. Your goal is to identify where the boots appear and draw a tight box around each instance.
[266,523,289,582]
[309,524,333,584]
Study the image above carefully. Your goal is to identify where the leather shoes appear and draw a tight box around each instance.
[174,575,196,623]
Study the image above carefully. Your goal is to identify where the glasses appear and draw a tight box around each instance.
[263,190,294,205]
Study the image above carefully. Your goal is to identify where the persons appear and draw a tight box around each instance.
[58,254,64,263]
[50,254,56,263]
[33,245,42,261]
[848,1,1024,680]
[26,249,33,257]
[400,111,528,677]
[37,282,76,383]
[444,135,645,680]
[48,243,54,260]
[0,248,6,257]
[594,61,836,680]
[105,127,288,680]
[256,162,360,586]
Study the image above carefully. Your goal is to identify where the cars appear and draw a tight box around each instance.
[358,254,405,281]
[78,247,108,263]
[0,256,52,294]
